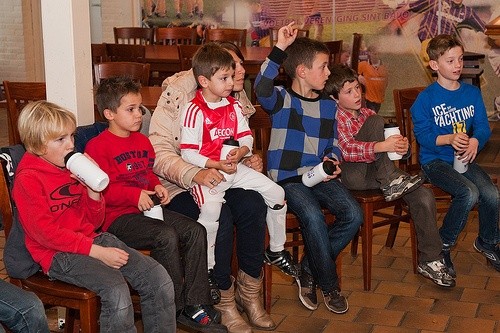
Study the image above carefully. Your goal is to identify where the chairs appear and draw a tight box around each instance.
[0,24,450,332]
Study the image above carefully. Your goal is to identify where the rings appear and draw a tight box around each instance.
[210,179,216,185]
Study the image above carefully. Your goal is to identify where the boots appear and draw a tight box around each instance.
[234,267,277,331]
[213,274,252,333]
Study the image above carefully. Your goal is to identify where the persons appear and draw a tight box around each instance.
[84,77,229,333]
[340,46,388,113]
[255,21,364,314]
[324,63,455,288]
[147,40,276,333]
[0,278,49,333]
[180,43,302,306]
[12,101,175,333]
[411,34,500,278]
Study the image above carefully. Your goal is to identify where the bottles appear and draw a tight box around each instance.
[218,136,240,181]
[302,158,336,188]
[143,194,163,222]
[64,151,109,192]
[454,149,469,174]
[383,119,402,161]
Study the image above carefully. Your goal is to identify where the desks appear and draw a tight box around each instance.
[431,53,487,88]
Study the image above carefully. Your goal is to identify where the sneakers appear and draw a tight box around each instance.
[264,244,297,277]
[473,236,500,272]
[382,175,426,202]
[208,268,221,305]
[416,258,456,287]
[295,269,317,310]
[177,304,228,333]
[442,246,457,278]
[320,288,349,313]
